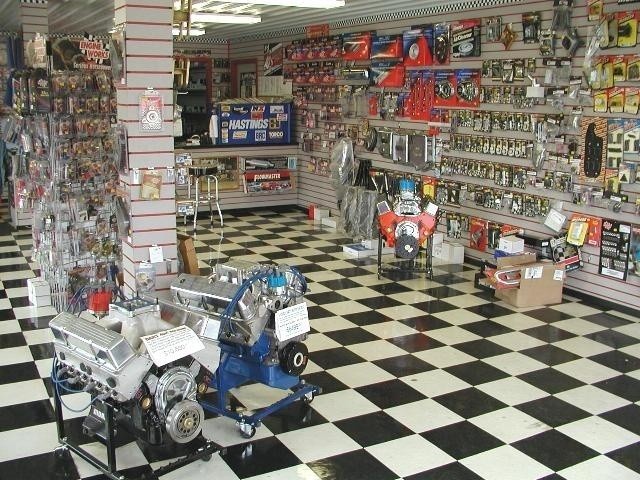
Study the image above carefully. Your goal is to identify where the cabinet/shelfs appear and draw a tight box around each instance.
[177,148,298,214]
[179,58,232,136]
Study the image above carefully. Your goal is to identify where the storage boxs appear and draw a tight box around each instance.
[432,233,443,256]
[448,243,464,264]
[178,233,199,275]
[499,236,524,253]
[322,217,340,228]
[440,242,449,260]
[495,255,566,309]
[339,244,394,259]
[313,207,329,221]
[365,240,382,250]
